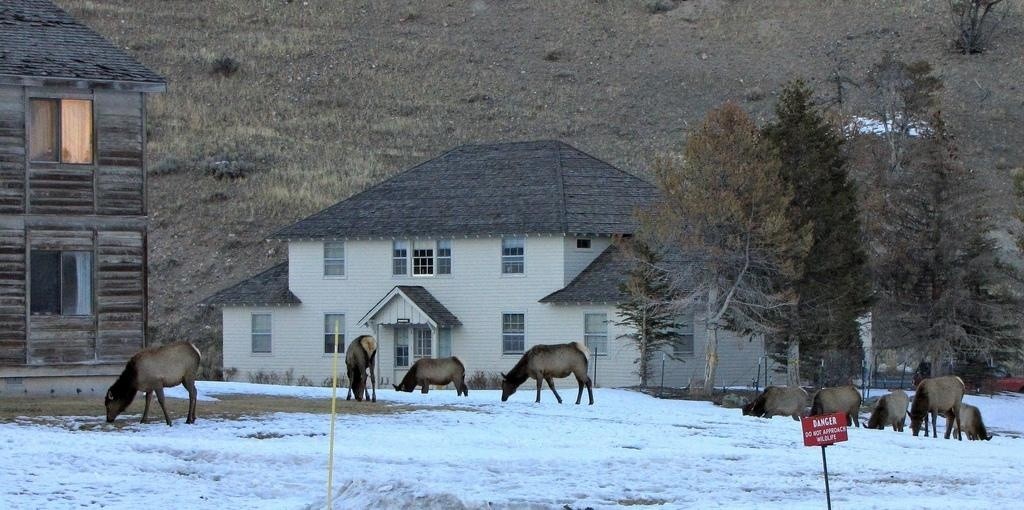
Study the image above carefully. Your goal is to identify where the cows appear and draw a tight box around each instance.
[865,388,911,433]
[936,401,994,441]
[103,338,202,427]
[344,334,379,404]
[905,374,966,441]
[749,384,812,423]
[391,356,469,398]
[498,340,595,407]
[802,385,863,427]
